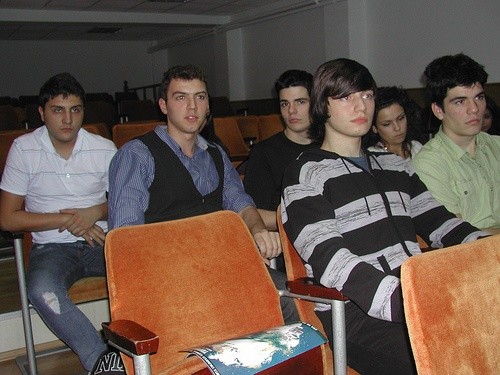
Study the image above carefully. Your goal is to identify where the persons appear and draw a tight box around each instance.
[481,102,500,135]
[243,70,313,230]
[366,86,423,162]
[0,73,118,375]
[406,52,500,229]
[280,57,494,375]
[108,63,301,325]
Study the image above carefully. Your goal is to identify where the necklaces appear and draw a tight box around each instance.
[384,144,388,151]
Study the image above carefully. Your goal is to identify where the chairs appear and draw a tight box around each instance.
[98,210,352,375]
[0,115,285,183]
[401,234,500,375]
[275,202,428,375]
[10,215,108,375]
